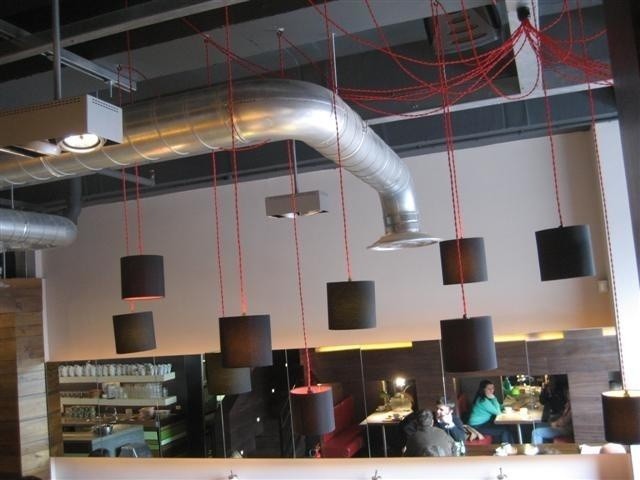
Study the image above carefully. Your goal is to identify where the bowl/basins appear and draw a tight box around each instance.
[393,414,400,419]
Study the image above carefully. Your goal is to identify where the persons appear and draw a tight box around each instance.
[502,376,512,396]
[531,385,574,444]
[403,409,457,457]
[466,380,515,445]
[403,396,465,442]
[539,375,567,422]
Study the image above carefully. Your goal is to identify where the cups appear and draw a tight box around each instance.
[61,362,172,377]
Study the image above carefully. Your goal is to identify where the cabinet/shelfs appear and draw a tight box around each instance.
[59,371,177,408]
